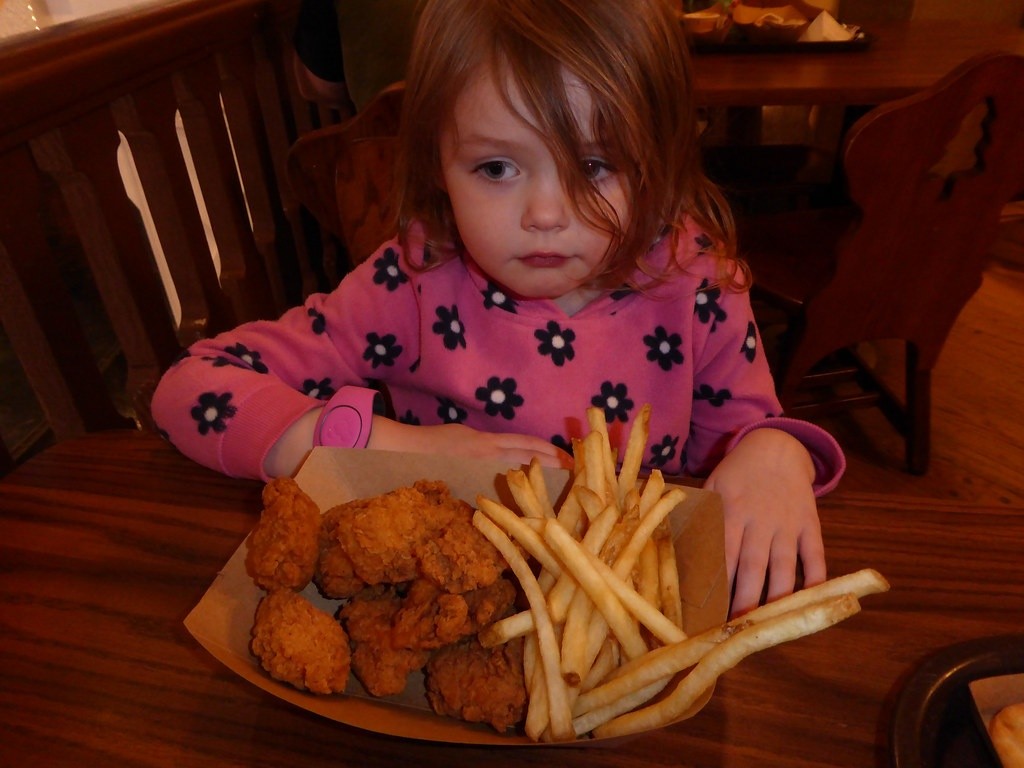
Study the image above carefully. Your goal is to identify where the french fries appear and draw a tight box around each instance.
[473,402,890,742]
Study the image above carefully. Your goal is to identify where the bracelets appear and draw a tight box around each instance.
[310,383,387,448]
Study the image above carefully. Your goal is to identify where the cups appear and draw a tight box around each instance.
[683,12,720,45]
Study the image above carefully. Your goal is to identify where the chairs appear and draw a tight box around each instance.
[735,50,1024,480]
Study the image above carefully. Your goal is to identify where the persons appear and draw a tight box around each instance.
[148,0,846,619]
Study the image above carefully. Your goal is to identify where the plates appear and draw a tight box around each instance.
[889,633,1024,768]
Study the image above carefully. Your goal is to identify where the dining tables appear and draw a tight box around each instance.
[0,431,1024,768]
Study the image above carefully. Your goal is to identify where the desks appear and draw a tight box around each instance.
[689,22,1024,143]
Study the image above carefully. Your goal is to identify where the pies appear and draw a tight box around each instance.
[990,702,1024,768]
